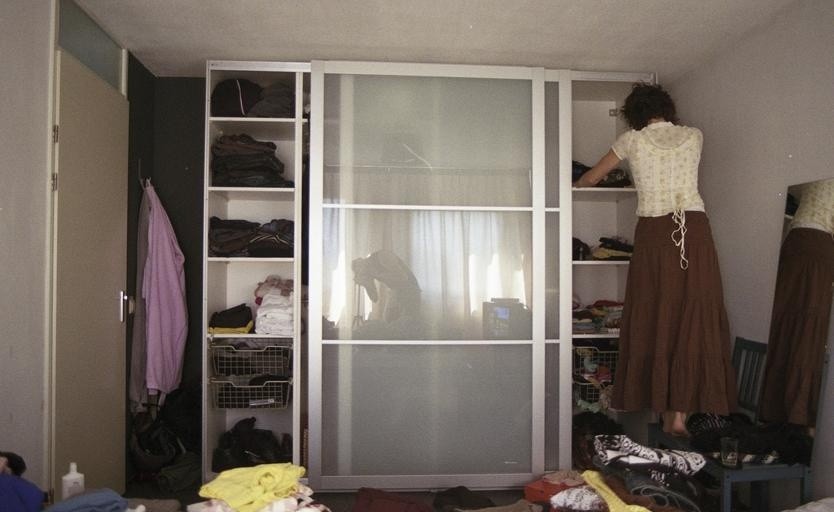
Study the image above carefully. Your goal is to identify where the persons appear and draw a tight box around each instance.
[577,82,740,435]
[349,248,421,321]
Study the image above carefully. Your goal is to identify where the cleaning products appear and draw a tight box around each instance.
[61,462,84,500]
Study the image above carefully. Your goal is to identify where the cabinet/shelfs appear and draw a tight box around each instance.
[200,58,660,491]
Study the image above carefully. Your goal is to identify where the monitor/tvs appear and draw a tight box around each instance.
[483,302,531,340]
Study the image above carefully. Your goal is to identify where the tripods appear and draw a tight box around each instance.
[351,284,364,327]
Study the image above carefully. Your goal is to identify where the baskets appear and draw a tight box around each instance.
[210,339,290,375]
[211,379,291,410]
[577,382,600,403]
[574,346,619,382]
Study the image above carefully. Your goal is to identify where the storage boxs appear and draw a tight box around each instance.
[525,477,577,504]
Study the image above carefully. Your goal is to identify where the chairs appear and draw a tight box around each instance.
[648,335,772,484]
[696,331,830,511]
